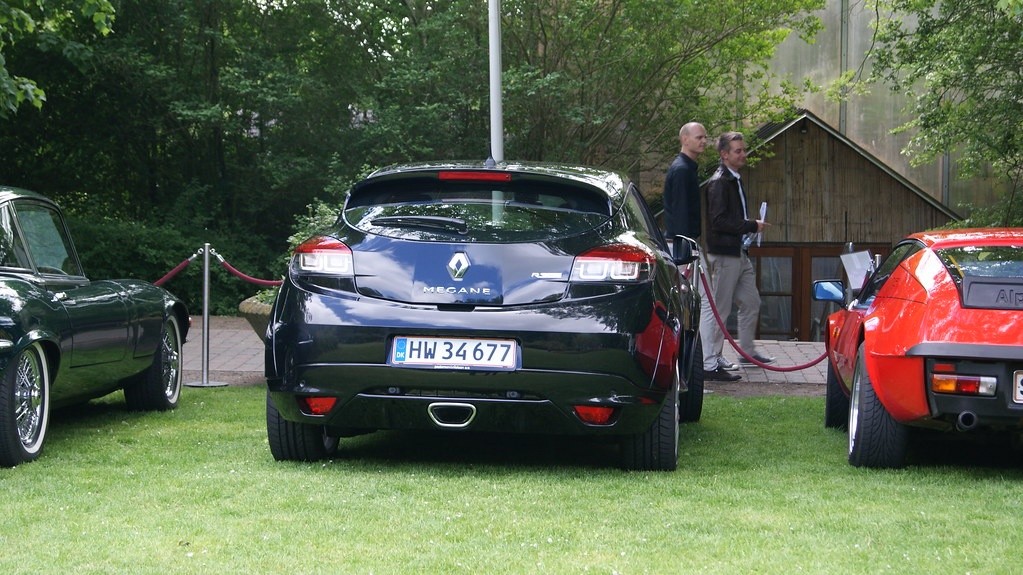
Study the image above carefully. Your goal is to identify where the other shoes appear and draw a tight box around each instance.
[703,367,742,381]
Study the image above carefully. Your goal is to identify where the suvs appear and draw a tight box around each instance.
[263,157,702,472]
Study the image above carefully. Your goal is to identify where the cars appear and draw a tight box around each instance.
[810,227,1023,472]
[0,185,194,470]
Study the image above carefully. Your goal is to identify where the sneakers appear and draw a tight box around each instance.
[718,355,738,369]
[738,354,776,368]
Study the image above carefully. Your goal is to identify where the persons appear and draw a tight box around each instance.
[705,131,778,371]
[665,123,742,382]
